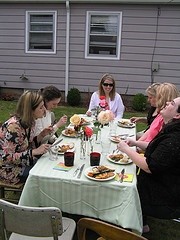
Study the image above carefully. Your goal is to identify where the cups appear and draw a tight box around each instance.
[63,152,75,166]
[90,151,101,166]
[48,145,58,161]
[109,122,117,136]
[80,141,87,159]
[101,139,111,155]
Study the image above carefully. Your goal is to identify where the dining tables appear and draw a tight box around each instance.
[17,115,143,236]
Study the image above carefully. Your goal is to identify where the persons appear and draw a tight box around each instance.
[130,83,161,138]
[135,82,180,154]
[29,85,67,144]
[117,96,180,239]
[86,74,124,119]
[0,89,54,182]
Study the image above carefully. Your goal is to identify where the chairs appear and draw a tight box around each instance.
[76,218,148,240]
[0,199,76,240]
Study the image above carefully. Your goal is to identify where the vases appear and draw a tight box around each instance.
[80,141,92,159]
[100,127,112,154]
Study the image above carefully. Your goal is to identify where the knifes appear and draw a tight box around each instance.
[46,138,63,150]
[120,168,125,183]
[77,164,85,179]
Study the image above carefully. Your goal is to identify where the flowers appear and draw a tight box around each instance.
[71,114,84,126]
[99,95,110,110]
[81,127,94,141]
[97,110,115,128]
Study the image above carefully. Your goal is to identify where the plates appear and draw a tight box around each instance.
[108,136,129,142]
[105,154,133,164]
[85,167,116,181]
[62,130,81,137]
[50,141,76,154]
[117,122,135,128]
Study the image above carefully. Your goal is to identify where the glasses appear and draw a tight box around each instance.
[102,82,114,87]
